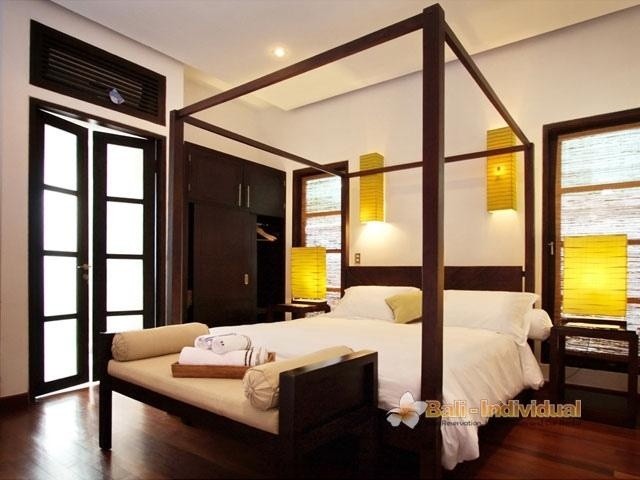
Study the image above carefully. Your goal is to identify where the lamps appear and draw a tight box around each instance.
[562,234,627,331]
[359,152,385,223]
[486,127,518,214]
[290,246,328,305]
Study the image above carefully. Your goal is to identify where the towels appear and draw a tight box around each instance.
[212,334,252,354]
[179,346,269,367]
[193,330,236,350]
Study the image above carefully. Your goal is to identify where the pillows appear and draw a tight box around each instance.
[112,321,209,362]
[383,291,422,324]
[242,345,353,411]
[443,286,539,349]
[527,309,554,341]
[329,284,420,323]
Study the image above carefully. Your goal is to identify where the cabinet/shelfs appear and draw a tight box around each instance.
[184,140,287,328]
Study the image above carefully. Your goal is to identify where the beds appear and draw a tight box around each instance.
[95,3,536,480]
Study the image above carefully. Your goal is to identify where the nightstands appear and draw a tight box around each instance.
[275,302,331,322]
[549,321,640,429]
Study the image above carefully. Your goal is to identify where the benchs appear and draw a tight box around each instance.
[97,323,379,480]
